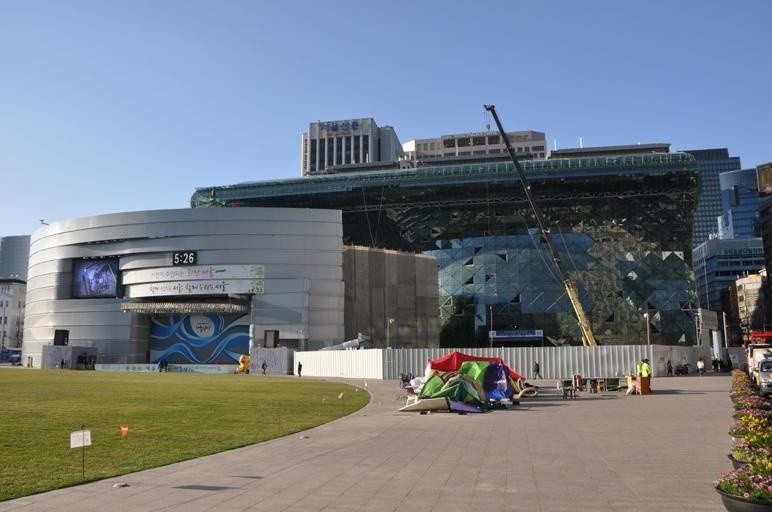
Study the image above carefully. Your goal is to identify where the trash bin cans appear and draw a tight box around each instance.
[27,357,33,368]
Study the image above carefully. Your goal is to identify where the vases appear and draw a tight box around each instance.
[715,487,771,511]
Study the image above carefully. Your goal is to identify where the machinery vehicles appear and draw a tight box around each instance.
[483,104,598,346]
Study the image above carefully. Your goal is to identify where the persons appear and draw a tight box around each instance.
[61,359,64,369]
[297,362,302,377]
[531,361,542,380]
[696,357,705,376]
[666,360,673,376]
[158,359,163,373]
[162,358,169,372]
[711,357,734,371]
[641,358,653,392]
[636,359,644,375]
[261,360,268,375]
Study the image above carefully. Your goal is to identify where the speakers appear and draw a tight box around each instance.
[54,330,69,345]
[264,330,279,348]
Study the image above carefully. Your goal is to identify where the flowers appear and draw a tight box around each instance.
[714,367,771,502]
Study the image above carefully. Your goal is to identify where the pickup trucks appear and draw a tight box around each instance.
[753,358,772,396]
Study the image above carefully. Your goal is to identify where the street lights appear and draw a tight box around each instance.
[388,319,395,347]
[644,313,650,345]
